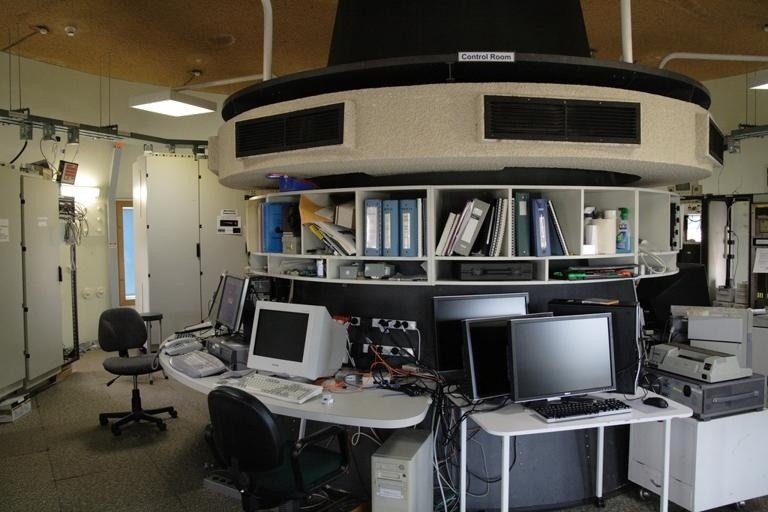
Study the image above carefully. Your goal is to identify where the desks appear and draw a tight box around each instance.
[443,383,693,512]
[156,327,437,439]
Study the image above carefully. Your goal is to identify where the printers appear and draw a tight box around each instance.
[650,342,753,384]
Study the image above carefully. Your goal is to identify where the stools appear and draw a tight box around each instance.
[140,312,163,352]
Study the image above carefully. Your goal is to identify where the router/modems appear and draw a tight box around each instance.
[362,373,390,387]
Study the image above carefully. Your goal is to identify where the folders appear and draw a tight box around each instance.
[398,201,418,256]
[454,199,490,255]
[381,200,398,257]
[518,190,532,256]
[533,196,550,257]
[364,199,381,257]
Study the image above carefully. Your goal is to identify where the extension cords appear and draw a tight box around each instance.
[363,343,419,359]
[370,317,419,330]
[343,315,361,325]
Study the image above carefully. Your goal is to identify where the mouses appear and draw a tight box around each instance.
[644,397,668,408]
[320,393,332,407]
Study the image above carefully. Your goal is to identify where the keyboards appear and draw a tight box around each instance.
[528,396,634,422]
[168,349,224,378]
[232,374,324,404]
[175,331,194,340]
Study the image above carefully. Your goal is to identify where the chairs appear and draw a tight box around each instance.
[98,307,178,436]
[203,385,350,512]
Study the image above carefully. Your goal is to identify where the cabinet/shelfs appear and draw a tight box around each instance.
[430,184,681,286]
[144,153,242,346]
[627,389,768,512]
[242,184,430,287]
[21,175,62,393]
[0,165,26,404]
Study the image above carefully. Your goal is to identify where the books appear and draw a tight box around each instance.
[417,199,427,258]
[482,198,507,257]
[307,222,356,256]
[258,201,296,252]
[435,201,473,256]
[512,198,516,256]
[547,200,569,256]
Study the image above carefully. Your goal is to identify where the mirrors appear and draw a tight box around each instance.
[59,213,80,365]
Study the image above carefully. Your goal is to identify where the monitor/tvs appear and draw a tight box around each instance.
[509,311,616,402]
[216,274,250,332]
[463,310,556,403]
[433,291,529,374]
[246,300,347,380]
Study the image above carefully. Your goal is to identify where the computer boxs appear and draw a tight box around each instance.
[370,429,435,512]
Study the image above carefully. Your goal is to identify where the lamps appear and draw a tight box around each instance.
[127,88,217,118]
[748,80,768,90]
